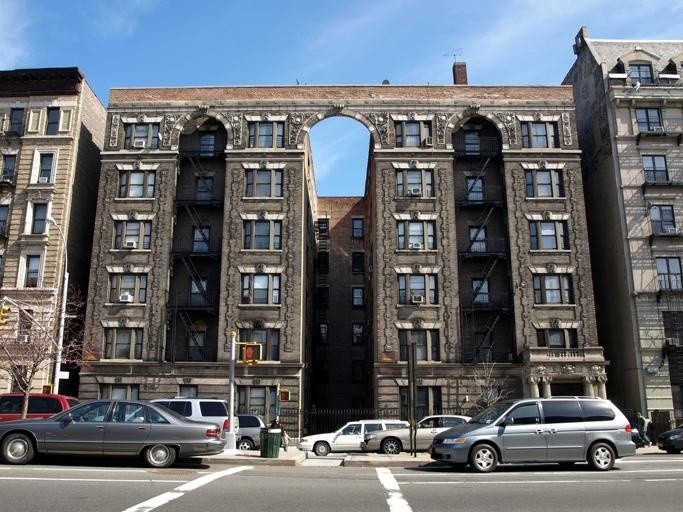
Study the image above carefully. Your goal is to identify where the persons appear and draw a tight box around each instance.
[281,430,293,452]
[270,414,284,435]
[635,411,653,448]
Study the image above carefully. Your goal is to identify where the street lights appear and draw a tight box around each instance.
[45,215,78,395]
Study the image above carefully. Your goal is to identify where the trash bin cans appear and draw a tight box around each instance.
[260,428,282,458]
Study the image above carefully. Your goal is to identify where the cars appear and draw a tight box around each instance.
[0,392,267,468]
[654,424,683,454]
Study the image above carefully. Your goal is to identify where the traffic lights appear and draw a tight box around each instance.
[243,344,262,365]
[279,389,290,402]
[0,302,10,327]
[42,384,53,394]
